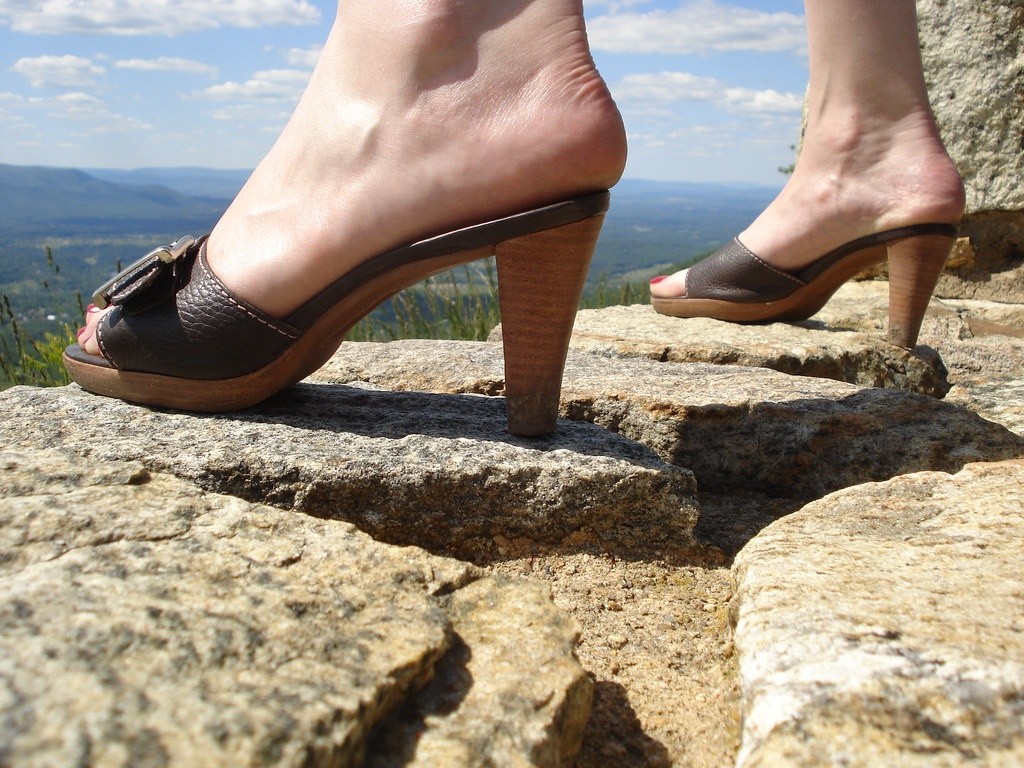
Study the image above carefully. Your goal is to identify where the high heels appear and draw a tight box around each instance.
[60,188,610,440]
[652,221,957,347]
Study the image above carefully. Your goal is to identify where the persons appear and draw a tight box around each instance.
[61,1,969,439]
